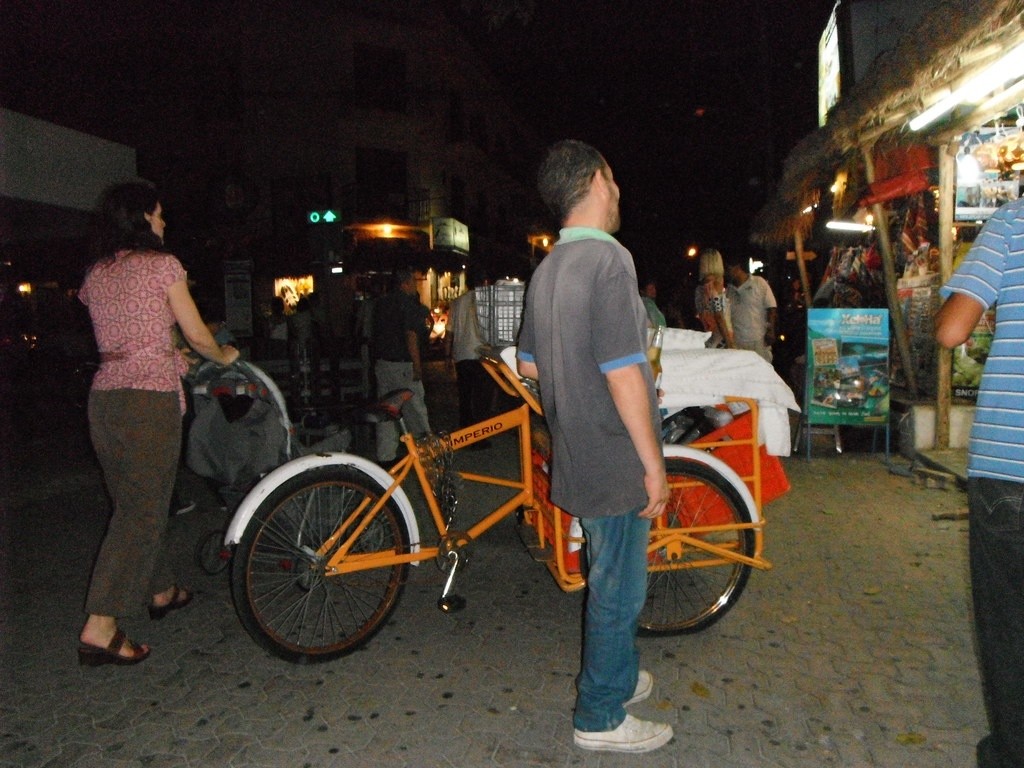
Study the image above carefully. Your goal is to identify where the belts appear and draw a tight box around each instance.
[377,356,414,362]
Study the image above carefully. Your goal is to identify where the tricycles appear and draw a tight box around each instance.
[221,353,774,664]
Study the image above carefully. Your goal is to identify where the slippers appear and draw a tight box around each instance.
[149,583,192,620]
[76,628,150,666]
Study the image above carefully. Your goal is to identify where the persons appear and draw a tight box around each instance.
[930,194,1024,768]
[634,247,818,396]
[78,177,241,665]
[515,140,674,756]
[166,251,497,517]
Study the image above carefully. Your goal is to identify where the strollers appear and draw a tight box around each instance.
[177,354,372,592]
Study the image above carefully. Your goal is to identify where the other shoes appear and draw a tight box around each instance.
[177,500,196,515]
[574,715,674,753]
[473,440,492,449]
[622,670,653,707]
